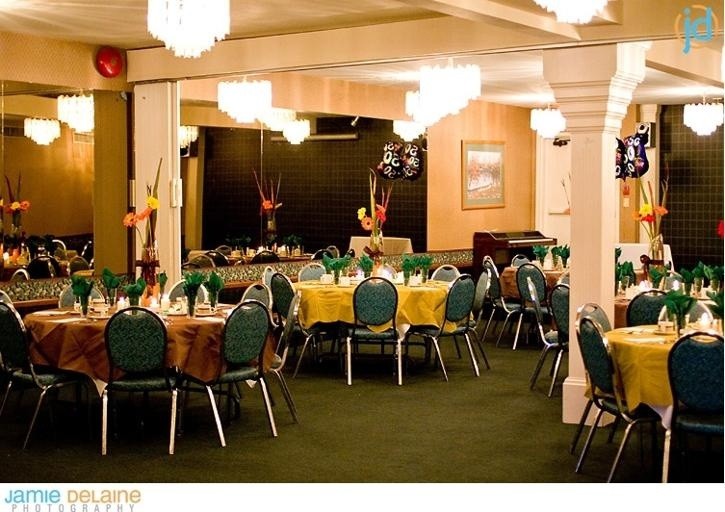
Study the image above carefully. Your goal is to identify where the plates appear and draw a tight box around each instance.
[70,299,215,322]
[623,327,721,345]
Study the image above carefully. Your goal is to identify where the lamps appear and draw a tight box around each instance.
[682,103,724,136]
[179,126,200,149]
[218,80,309,145]
[533,0,608,26]
[147,0,230,59]
[530,108,566,141]
[393,64,481,144]
[24,117,61,145]
[270,134,357,141]
[57,92,95,134]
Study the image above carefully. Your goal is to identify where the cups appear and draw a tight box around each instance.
[231,248,300,256]
[626,283,716,300]
[319,275,424,287]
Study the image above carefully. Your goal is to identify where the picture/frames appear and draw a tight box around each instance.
[461,138,507,211]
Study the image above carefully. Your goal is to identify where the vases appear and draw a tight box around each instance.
[370,231,383,265]
[267,212,279,244]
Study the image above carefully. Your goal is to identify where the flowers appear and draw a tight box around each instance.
[122,157,163,258]
[3,168,31,226]
[252,167,283,220]
[358,167,394,248]
[632,153,669,258]
